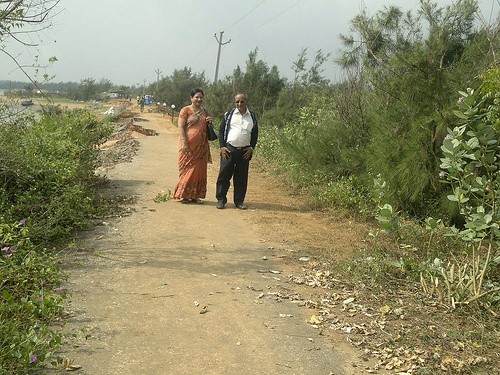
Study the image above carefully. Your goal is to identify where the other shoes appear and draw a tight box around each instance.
[235,203,248,209]
[182,198,204,204]
[216,198,227,209]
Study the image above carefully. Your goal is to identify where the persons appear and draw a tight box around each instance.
[216,91,258,209]
[172,88,212,205]
[125,93,146,113]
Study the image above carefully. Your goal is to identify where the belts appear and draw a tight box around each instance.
[228,143,250,150]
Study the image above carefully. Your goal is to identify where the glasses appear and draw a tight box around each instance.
[235,100,245,103]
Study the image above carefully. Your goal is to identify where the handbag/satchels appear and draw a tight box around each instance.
[203,107,218,141]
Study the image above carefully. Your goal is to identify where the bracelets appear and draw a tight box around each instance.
[250,147,254,151]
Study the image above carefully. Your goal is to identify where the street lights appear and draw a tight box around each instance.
[162,102,167,115]
[171,103,175,123]
[157,101,161,113]
